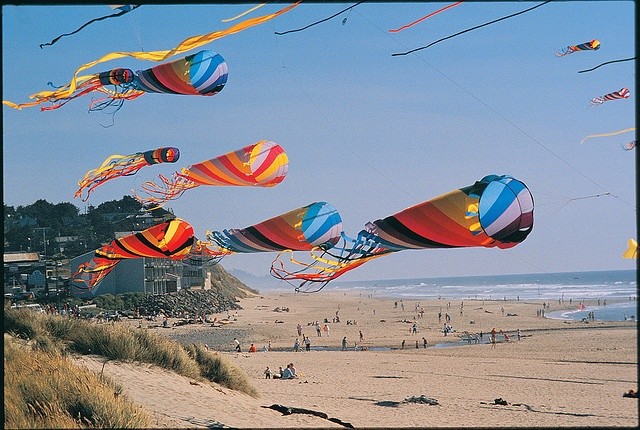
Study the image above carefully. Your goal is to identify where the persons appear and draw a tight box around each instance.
[536,295,639,325]
[264,365,271,380]
[415,341,418,349]
[359,331,365,342]
[294,311,340,352]
[282,365,295,379]
[233,337,241,352]
[268,340,273,351]
[288,363,296,379]
[273,366,284,379]
[341,336,348,351]
[400,340,406,350]
[393,299,452,336]
[248,344,256,353]
[467,327,522,349]
[622,388,640,398]
[422,337,427,348]
[342,293,386,325]
[455,295,520,325]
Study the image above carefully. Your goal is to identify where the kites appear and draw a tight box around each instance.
[88,50,229,129]
[74,146,181,203]
[178,201,343,268]
[68,217,195,295]
[131,139,289,213]
[587,87,630,108]
[2,67,134,112]
[269,173,535,295]
[622,238,640,259]
[38,4,151,50]
[555,39,601,58]
[621,139,640,151]
